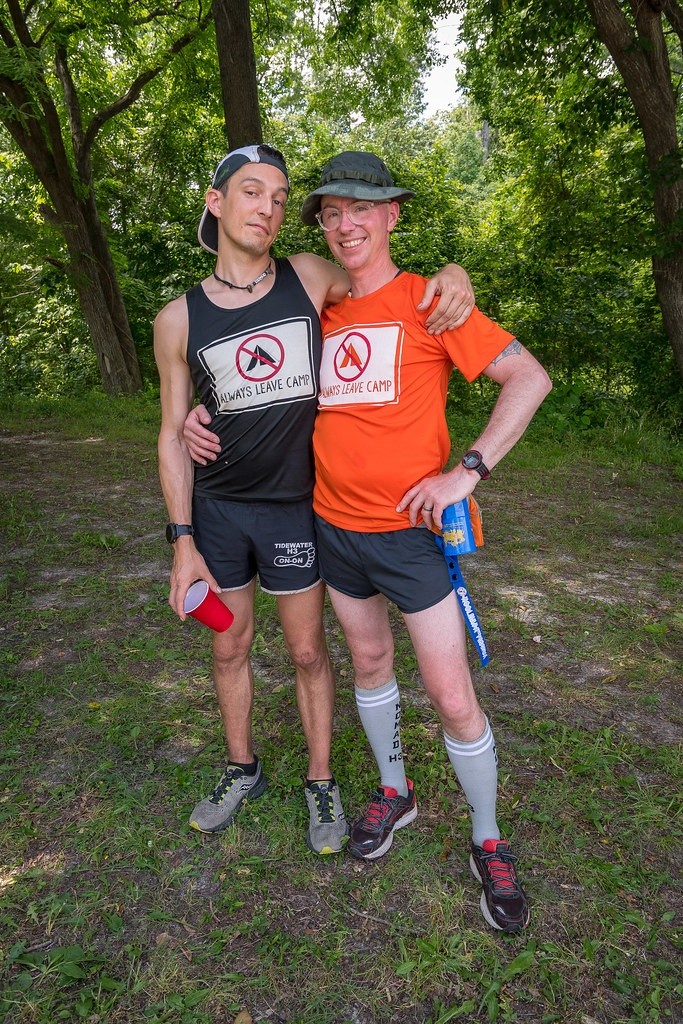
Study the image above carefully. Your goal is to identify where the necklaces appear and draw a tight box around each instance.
[214,259,273,292]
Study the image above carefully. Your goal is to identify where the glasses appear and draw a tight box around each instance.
[314,199,392,232]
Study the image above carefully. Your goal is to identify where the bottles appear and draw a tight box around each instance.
[440,497,478,557]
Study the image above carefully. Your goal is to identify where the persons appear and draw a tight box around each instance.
[152,143,476,855]
[183,151,553,933]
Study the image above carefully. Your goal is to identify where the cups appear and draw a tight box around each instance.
[181,579,235,634]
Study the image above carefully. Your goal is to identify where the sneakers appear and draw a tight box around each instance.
[304,772,348,856]
[188,756,266,833]
[347,778,418,859]
[469,835,531,931]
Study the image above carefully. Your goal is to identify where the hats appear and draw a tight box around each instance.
[301,151,415,228]
[198,145,289,257]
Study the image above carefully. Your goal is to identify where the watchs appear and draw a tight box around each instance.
[166,523,195,544]
[462,449,491,480]
